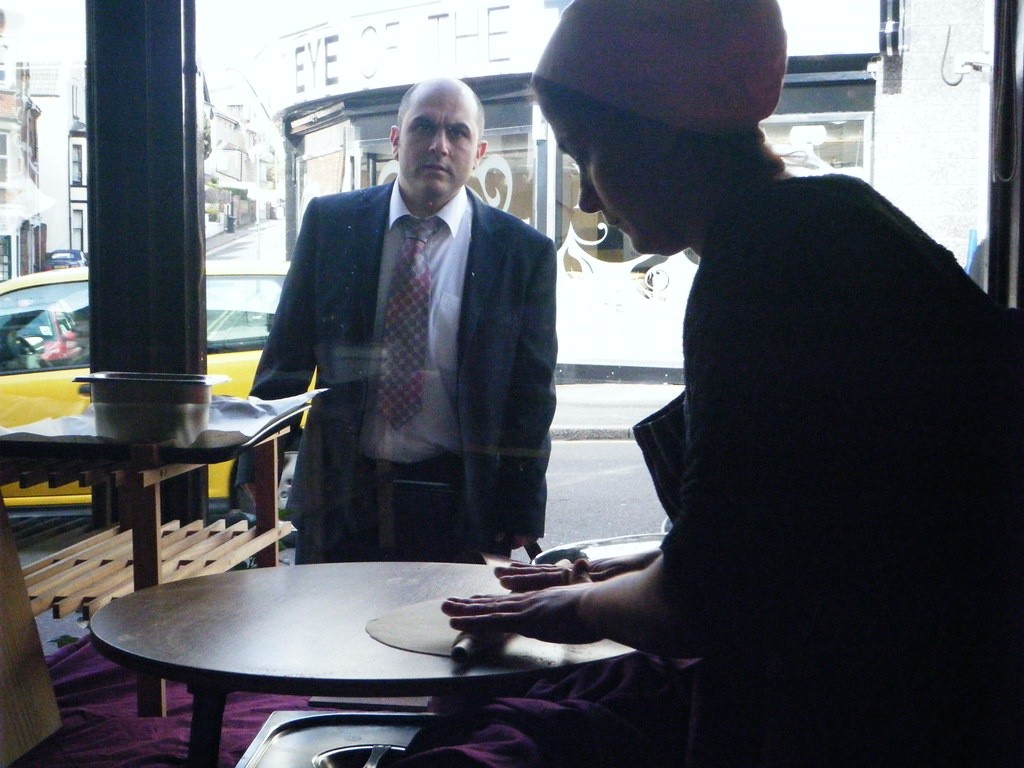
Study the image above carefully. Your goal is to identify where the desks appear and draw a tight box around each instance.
[89,562,637,768]
[0,421,293,719]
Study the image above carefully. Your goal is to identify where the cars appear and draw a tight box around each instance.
[0,266,317,508]
[44,250,87,272]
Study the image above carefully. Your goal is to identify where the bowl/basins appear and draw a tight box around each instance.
[70,369,229,450]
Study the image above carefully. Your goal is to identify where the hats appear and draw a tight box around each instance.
[533,0,788,135]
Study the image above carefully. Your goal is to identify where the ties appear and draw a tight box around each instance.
[374,216,446,431]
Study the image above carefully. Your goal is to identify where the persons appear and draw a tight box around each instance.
[397,0,1024,768]
[235,78,557,565]
[68,305,89,365]
